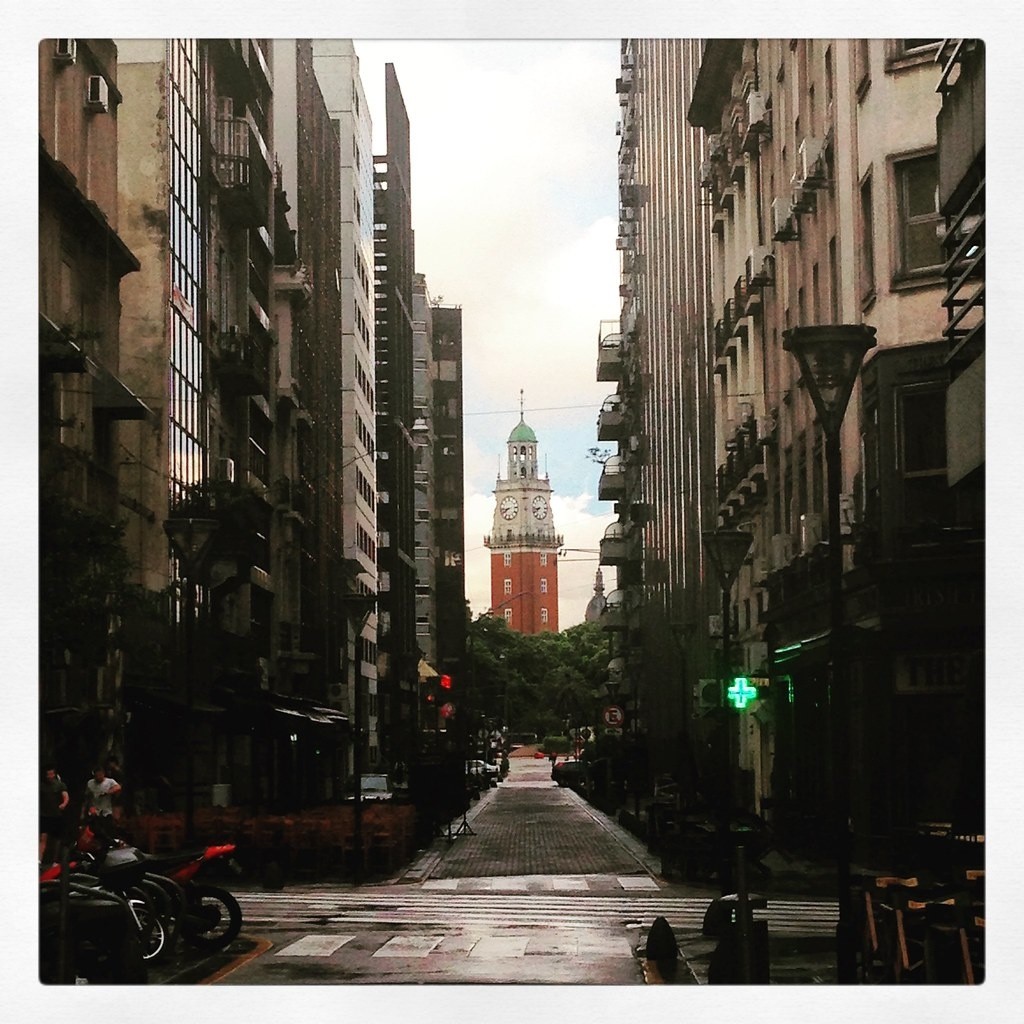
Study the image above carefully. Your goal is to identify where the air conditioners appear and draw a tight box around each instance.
[790,173,808,209]
[767,533,794,573]
[770,197,788,239]
[743,247,767,285]
[88,76,109,113]
[756,414,774,438]
[799,513,821,551]
[57,39,77,65]
[217,457,235,483]
[799,136,819,180]
[691,678,725,716]
[745,92,764,130]
[707,615,725,637]
[616,53,648,533]
[709,134,720,155]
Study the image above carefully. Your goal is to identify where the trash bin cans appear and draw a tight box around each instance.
[363,833,397,882]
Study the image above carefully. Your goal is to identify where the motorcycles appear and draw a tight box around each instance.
[40,806,243,967]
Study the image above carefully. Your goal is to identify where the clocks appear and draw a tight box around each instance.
[501,497,518,520]
[533,497,548,519]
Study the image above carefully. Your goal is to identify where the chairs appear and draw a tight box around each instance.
[860,871,984,984]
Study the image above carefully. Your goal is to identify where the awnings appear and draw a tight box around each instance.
[118,680,352,730]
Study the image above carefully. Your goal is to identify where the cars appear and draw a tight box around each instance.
[343,772,396,803]
[465,737,505,779]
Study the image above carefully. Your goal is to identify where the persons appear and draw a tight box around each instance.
[40,754,128,872]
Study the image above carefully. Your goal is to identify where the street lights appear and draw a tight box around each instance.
[702,530,754,891]
[341,591,382,885]
